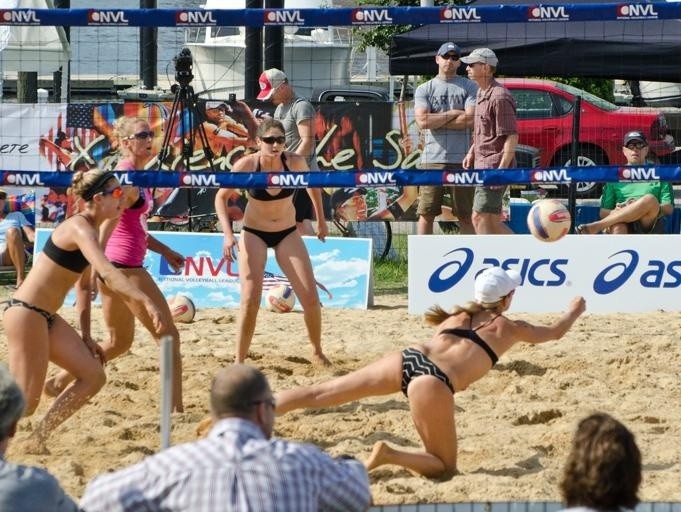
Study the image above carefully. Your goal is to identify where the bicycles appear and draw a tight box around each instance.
[159,219,393,268]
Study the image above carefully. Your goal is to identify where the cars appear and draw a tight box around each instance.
[496,78,674,199]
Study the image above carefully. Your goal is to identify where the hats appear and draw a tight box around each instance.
[624,131,648,147]
[256,68,286,100]
[438,42,461,58]
[206,101,228,110]
[459,48,497,67]
[474,268,521,304]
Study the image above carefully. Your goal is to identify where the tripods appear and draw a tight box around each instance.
[152,86,218,231]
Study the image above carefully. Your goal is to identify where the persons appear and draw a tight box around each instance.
[216,117,336,371]
[558,413,641,511]
[575,132,674,233]
[0,190,36,283]
[0,369,77,512]
[457,48,518,233]
[194,266,586,479]
[313,109,366,223]
[191,101,253,172]
[45,117,186,421]
[413,41,482,235]
[3,168,168,457]
[79,364,374,510]
[256,68,319,234]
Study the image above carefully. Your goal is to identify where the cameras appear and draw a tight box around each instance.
[173,48,194,87]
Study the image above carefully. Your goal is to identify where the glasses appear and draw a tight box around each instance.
[260,136,285,144]
[439,54,460,60]
[465,62,484,67]
[121,131,154,140]
[253,396,275,411]
[100,186,123,197]
[625,143,647,148]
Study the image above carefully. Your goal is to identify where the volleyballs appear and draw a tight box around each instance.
[266,284,296,313]
[527,200,571,243]
[167,296,195,323]
